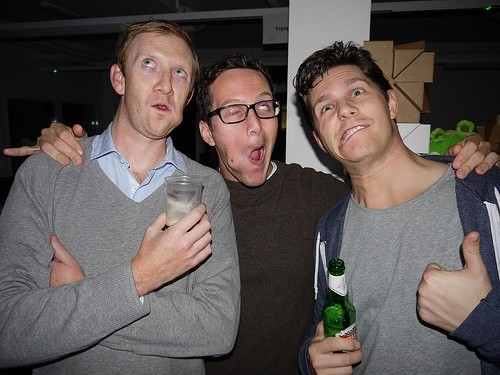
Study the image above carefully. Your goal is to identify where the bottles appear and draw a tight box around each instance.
[323,259,356,354]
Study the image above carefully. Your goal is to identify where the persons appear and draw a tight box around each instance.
[0,17,243,375]
[292,42,499,375]
[3,51,500,375]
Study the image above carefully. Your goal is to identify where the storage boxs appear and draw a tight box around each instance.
[361,40,436,157]
[476,114,500,155]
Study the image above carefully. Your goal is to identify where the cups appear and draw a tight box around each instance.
[164,176,203,227]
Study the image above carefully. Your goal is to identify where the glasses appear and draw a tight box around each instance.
[203,99,280,124]
